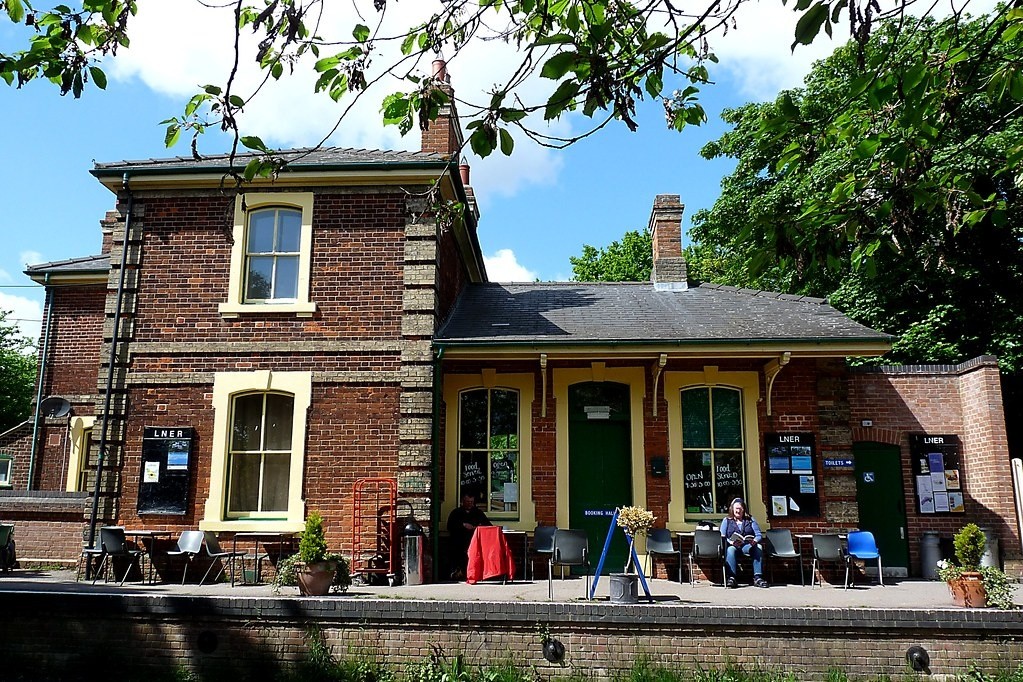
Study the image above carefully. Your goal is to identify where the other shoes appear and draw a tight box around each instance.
[727,577,738,589]
[753,576,768,587]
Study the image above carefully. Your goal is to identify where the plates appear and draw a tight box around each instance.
[502,530,515,532]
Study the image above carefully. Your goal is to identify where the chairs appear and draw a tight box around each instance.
[75,525,247,588]
[844,531,884,587]
[688,529,727,588]
[643,527,682,582]
[548,528,592,602]
[765,529,804,585]
[810,533,853,590]
[529,525,558,581]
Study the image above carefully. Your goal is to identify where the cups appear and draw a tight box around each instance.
[695,526,719,531]
[503,526,508,530]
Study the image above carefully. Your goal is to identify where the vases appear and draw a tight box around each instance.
[948,571,987,609]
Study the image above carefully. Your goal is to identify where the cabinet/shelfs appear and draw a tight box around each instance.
[348,475,398,584]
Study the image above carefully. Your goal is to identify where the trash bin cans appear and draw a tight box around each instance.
[398,523,424,586]
[976,527,1000,571]
[920,531,943,581]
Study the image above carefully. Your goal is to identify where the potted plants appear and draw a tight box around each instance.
[274,509,352,596]
[609,504,657,603]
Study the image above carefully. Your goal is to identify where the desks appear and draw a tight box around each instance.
[124,530,173,585]
[793,534,848,587]
[232,531,294,586]
[675,532,728,587]
[503,531,528,581]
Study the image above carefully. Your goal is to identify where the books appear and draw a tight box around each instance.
[730,531,754,546]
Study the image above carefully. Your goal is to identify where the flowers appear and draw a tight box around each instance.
[934,523,1021,611]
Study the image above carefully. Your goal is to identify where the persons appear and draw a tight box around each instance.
[719,498,769,588]
[447,491,493,579]
